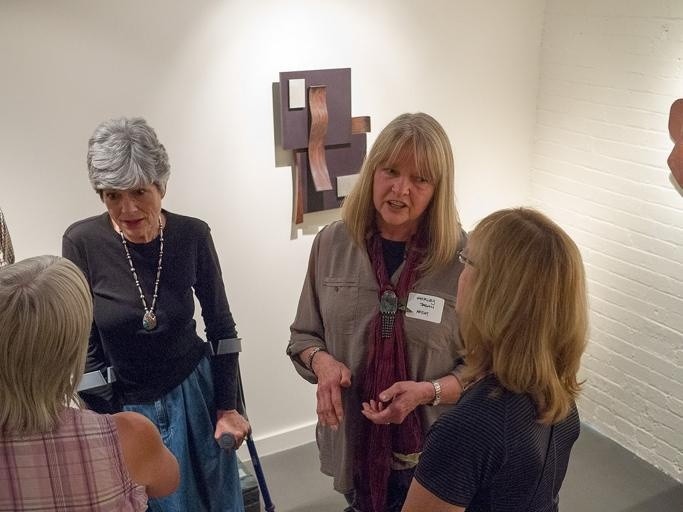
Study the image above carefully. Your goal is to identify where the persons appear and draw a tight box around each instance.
[52,118,252,511]
[398,208,590,512]
[1,252,182,511]
[286,112,483,512]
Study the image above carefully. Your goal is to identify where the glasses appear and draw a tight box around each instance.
[457,249,474,267]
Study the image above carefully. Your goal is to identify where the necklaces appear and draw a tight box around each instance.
[369,235,416,341]
[114,212,168,338]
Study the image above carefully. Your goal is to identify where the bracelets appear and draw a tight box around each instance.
[425,378,441,408]
[305,345,326,373]
